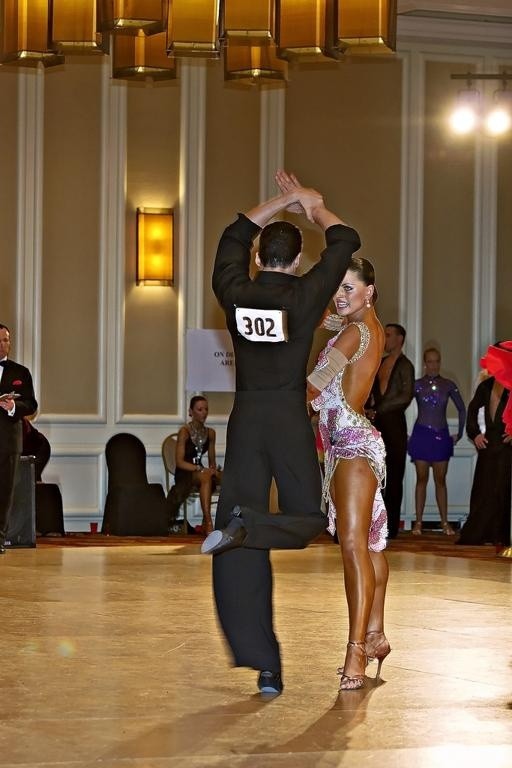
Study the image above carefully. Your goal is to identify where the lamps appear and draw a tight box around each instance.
[131,204,180,290]
[449,71,512,132]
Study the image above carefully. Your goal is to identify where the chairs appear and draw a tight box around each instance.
[158,432,220,535]
[96,430,174,535]
[28,430,67,540]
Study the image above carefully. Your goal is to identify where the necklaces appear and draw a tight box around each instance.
[190,422,207,442]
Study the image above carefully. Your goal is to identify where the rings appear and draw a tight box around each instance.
[3,406,5,409]
[4,404,6,407]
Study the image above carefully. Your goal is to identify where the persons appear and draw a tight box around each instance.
[199,166,364,696]
[452,375,512,547]
[366,321,416,540]
[305,254,399,690]
[0,322,41,554]
[175,395,223,536]
[407,344,468,534]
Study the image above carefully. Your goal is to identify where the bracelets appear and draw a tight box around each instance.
[372,406,377,416]
[193,464,205,472]
[322,315,345,333]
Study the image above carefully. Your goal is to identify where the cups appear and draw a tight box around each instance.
[90,522,98,534]
[195,524,201,534]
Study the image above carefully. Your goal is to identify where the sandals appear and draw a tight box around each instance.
[339,641,369,690]
[411,521,422,537]
[440,521,457,536]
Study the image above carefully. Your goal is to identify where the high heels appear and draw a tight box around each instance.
[201,519,214,537]
[256,667,283,693]
[201,504,253,555]
[336,631,392,679]
